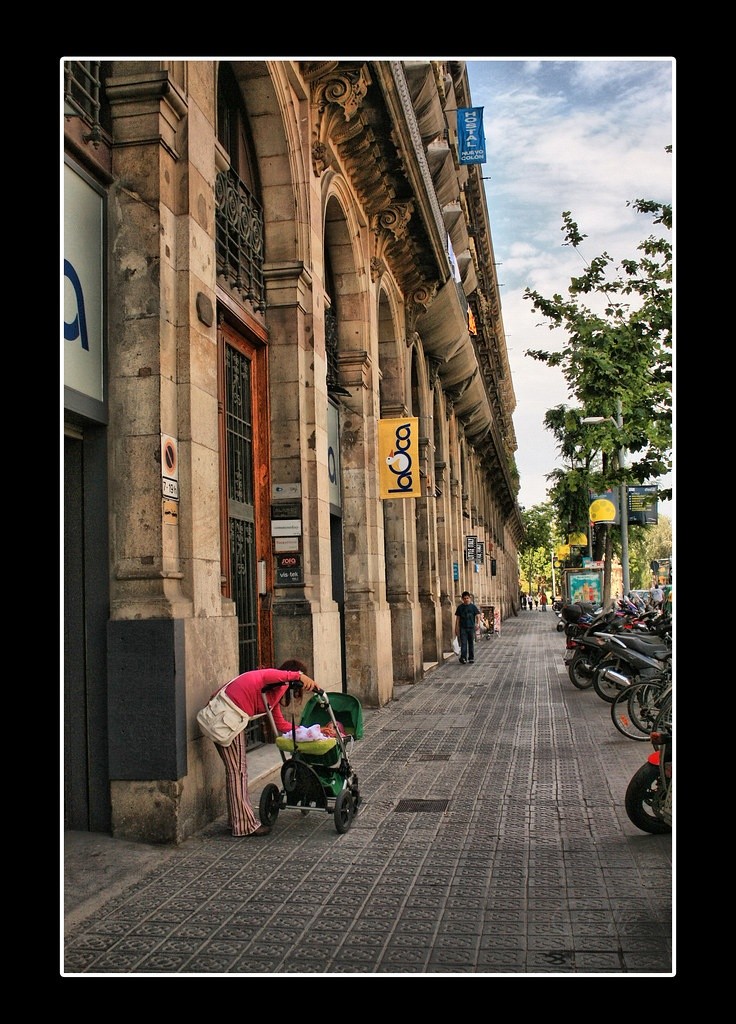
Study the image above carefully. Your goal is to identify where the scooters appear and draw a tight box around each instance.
[548,586,672,834]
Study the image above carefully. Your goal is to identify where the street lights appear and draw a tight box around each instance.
[578,415,632,598]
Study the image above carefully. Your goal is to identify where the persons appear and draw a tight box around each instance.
[455,591,481,664]
[326,721,341,736]
[205,659,320,837]
[650,583,672,614]
[522,591,548,612]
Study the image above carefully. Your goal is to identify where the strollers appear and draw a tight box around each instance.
[257,680,364,836]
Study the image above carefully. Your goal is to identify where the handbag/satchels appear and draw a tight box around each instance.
[453,636,460,656]
[196,690,249,747]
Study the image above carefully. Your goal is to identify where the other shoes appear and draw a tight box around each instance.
[227,824,233,830]
[469,659,474,663]
[249,826,270,835]
[459,657,467,664]
